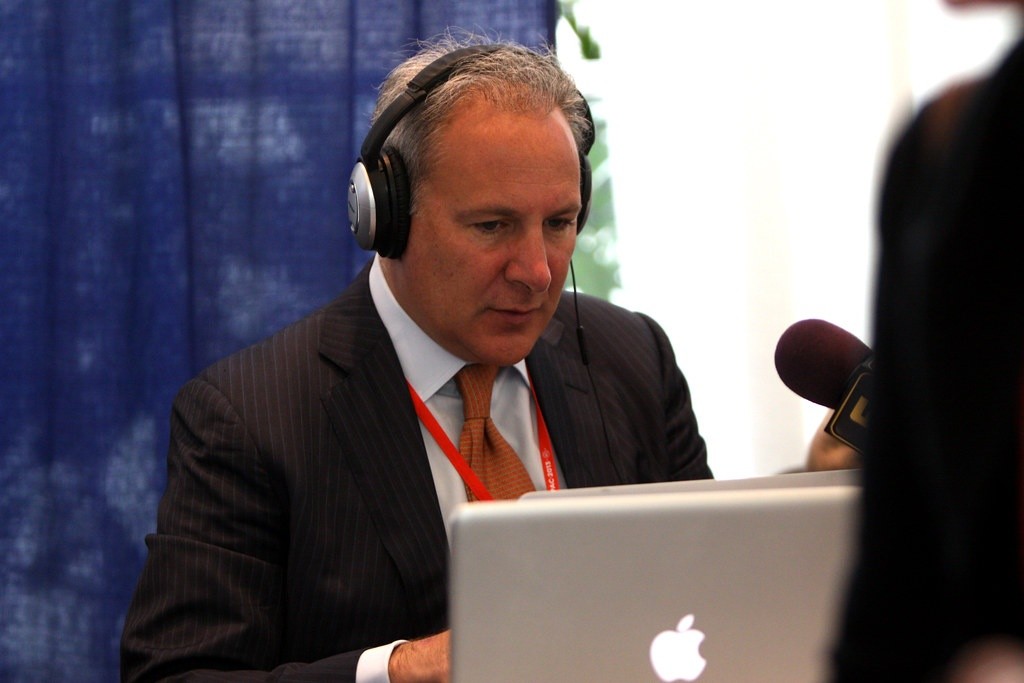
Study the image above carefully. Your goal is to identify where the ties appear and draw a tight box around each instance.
[453,363,536,502]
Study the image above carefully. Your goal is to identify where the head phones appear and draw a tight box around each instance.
[347,45,596,259]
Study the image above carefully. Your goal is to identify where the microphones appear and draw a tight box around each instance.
[774,319,873,458]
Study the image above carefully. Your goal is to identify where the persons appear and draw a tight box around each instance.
[825,1,1024,682]
[121,44,864,682]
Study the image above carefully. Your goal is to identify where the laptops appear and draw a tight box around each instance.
[449,488,862,683]
